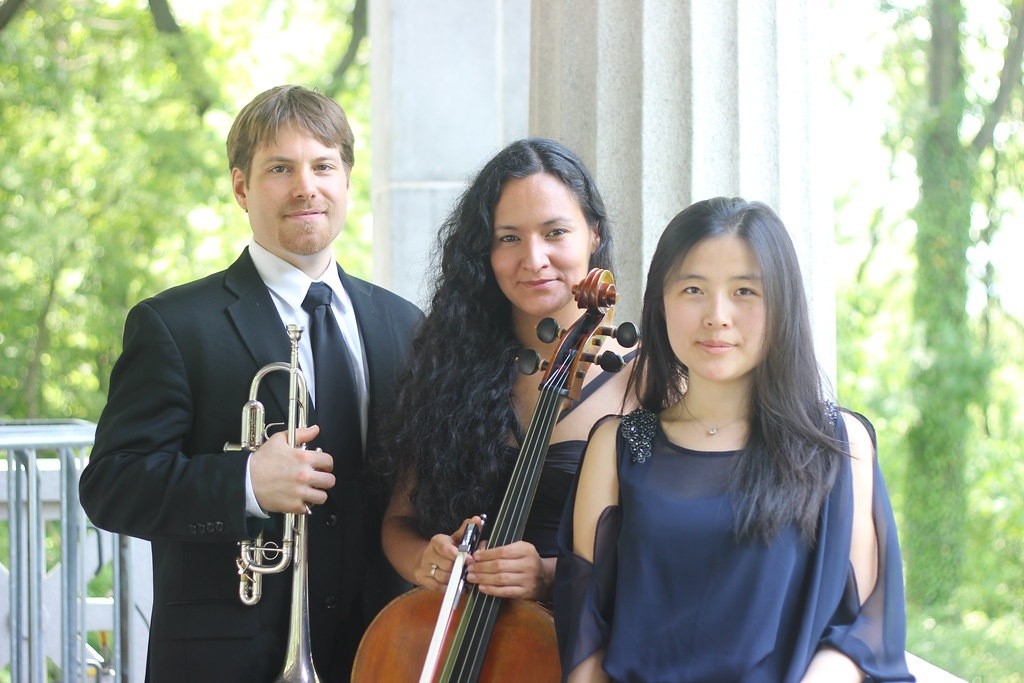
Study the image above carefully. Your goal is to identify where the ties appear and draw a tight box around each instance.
[301,284,367,683]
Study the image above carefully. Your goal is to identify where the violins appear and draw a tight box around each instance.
[348,264,640,683]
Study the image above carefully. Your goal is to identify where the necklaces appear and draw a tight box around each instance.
[684,393,743,434]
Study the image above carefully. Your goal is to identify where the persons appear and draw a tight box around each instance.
[380,139,649,610]
[78,84,426,682]
[547,198,916,683]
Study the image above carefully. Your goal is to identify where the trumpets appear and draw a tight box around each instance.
[221,324,327,683]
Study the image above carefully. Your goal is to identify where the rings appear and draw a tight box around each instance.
[430,565,437,575]
